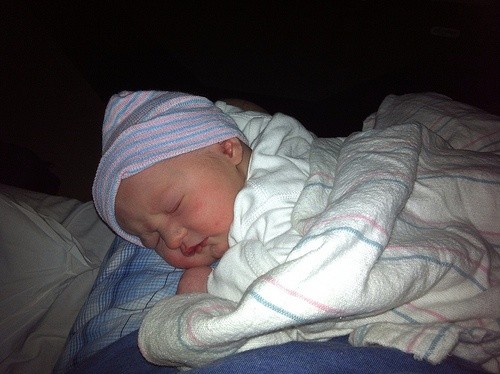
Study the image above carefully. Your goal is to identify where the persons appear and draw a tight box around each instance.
[91,91,314,306]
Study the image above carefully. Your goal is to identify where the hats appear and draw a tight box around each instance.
[91,91,250,248]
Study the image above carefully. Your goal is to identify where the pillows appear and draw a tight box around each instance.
[0,190,104,374]
